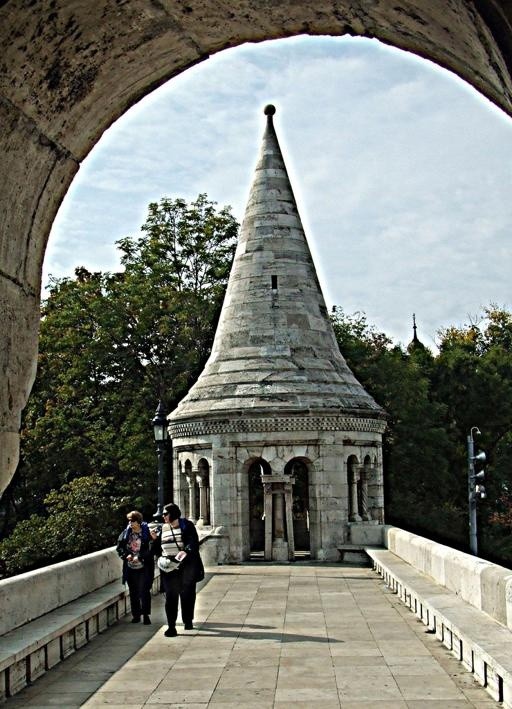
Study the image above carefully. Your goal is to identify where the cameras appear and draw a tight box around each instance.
[154,524,162,535]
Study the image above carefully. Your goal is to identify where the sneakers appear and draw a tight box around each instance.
[185,622,193,630]
[132,615,150,625]
[165,628,177,637]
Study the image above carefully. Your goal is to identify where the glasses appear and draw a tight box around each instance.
[163,512,169,516]
[129,519,136,522]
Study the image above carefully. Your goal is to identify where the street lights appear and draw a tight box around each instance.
[151,398,168,524]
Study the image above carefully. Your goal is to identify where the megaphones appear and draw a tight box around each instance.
[476,468,486,479]
[474,450,488,462]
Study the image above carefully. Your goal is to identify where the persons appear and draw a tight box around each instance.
[149,503,204,637]
[117,509,154,625]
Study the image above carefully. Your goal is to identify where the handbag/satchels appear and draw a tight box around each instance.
[157,555,180,572]
[128,555,144,568]
[183,558,204,585]
